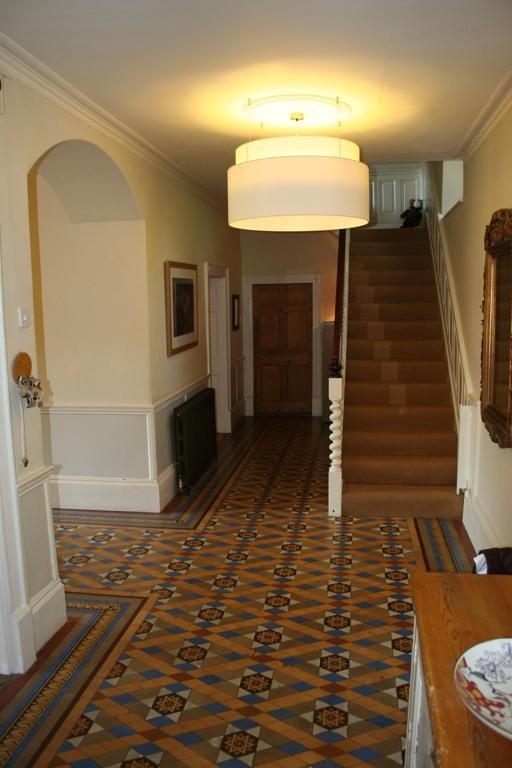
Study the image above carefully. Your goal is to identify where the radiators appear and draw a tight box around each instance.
[172,386,219,497]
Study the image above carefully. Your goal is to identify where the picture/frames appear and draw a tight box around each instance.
[163,259,199,358]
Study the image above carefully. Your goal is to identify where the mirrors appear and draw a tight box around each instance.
[478,206,512,450]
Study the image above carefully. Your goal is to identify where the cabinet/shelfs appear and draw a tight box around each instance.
[402,571,512,768]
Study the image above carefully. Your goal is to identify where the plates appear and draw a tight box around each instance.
[452,635,512,742]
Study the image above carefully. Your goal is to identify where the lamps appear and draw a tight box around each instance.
[225,92,372,234]
